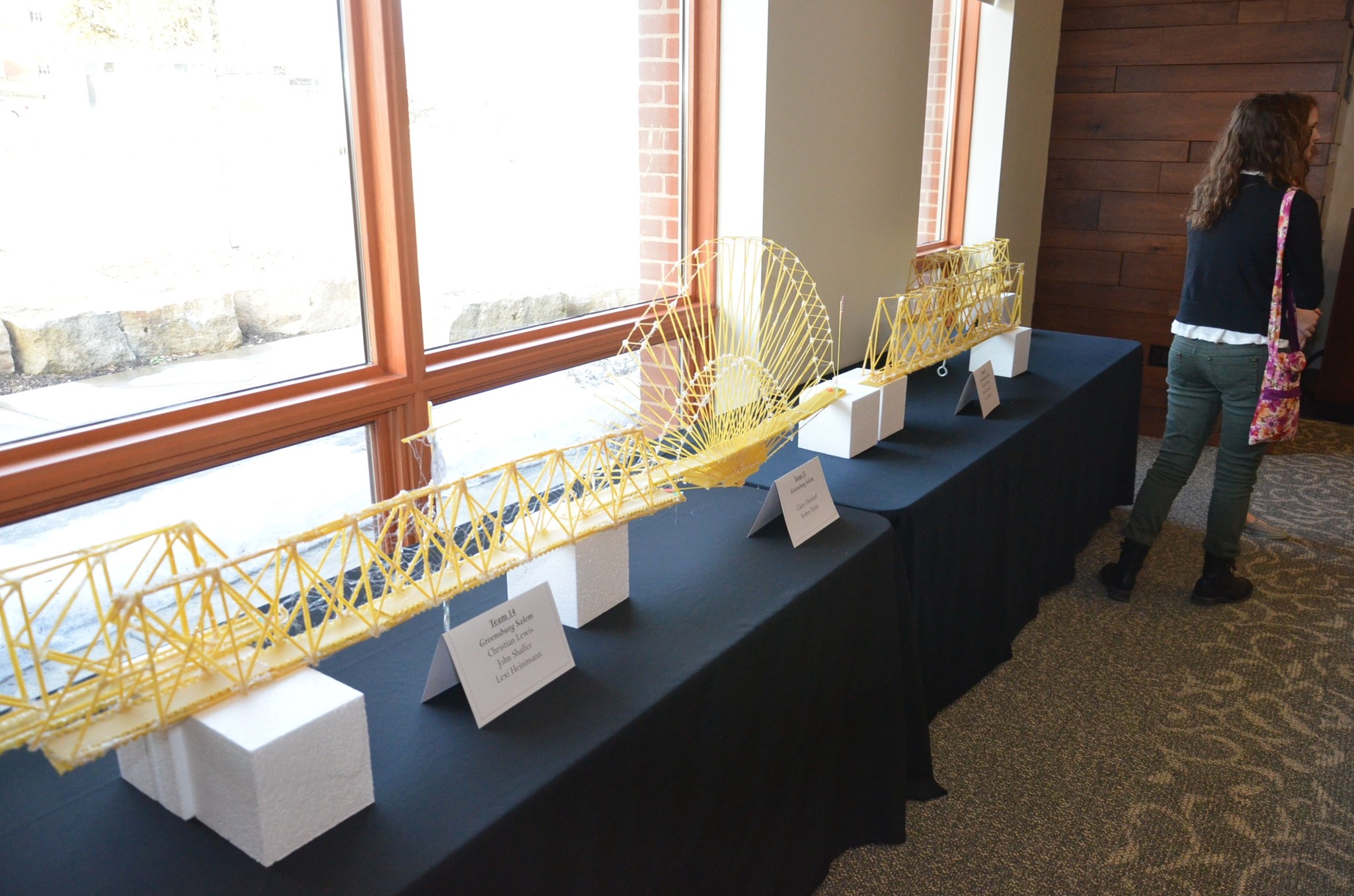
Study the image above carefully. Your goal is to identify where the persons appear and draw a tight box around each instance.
[1098,91,1326,607]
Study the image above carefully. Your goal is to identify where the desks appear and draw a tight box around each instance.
[0,327,1144,896]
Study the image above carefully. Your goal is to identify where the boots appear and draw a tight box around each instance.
[1193,551,1253,605]
[1101,538,1150,602]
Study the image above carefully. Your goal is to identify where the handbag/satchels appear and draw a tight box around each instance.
[1248,351,1306,446]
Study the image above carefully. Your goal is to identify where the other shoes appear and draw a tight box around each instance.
[1243,518,1289,539]
